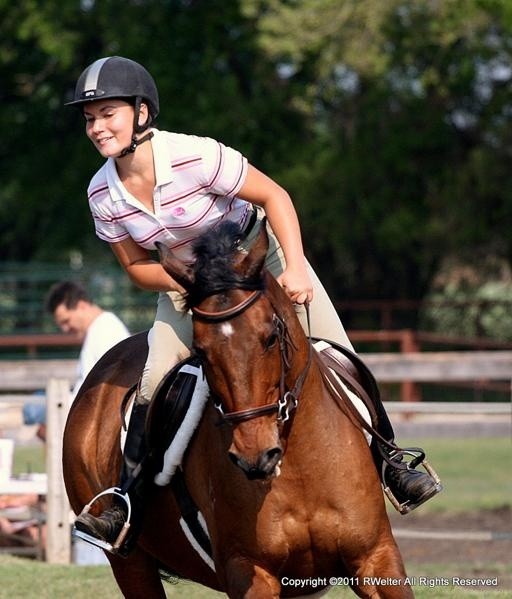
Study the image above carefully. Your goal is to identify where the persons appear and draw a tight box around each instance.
[60,56,440,561]
[41,277,137,398]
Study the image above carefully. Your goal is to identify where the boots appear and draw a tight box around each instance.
[75,501,134,559]
[374,439,443,502]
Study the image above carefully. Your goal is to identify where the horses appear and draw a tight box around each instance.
[60,214,418,599]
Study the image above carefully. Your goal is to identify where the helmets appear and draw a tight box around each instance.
[63,56,159,117]
